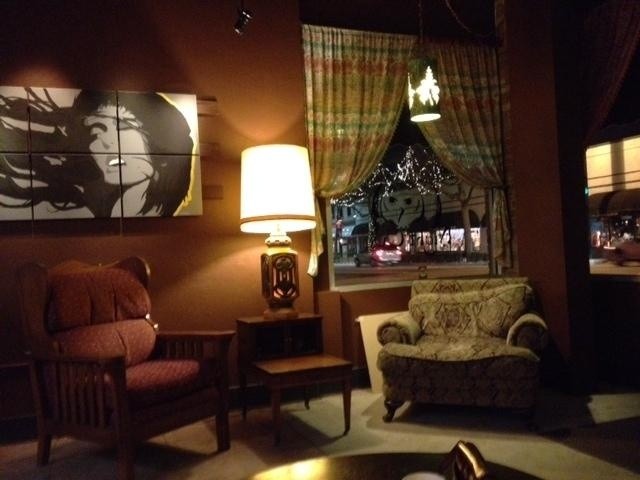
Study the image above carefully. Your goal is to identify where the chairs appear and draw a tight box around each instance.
[22,256,233,478]
[377,276,553,421]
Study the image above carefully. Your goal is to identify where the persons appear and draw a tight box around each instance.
[0,87,191,225]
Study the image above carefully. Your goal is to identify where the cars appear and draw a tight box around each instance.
[354,244,405,269]
[602,236,639,266]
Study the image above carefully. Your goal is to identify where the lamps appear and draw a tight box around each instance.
[238,144,319,325]
[401,1,444,123]
[232,0,256,36]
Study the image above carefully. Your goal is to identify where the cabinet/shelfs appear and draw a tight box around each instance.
[237,314,324,413]
